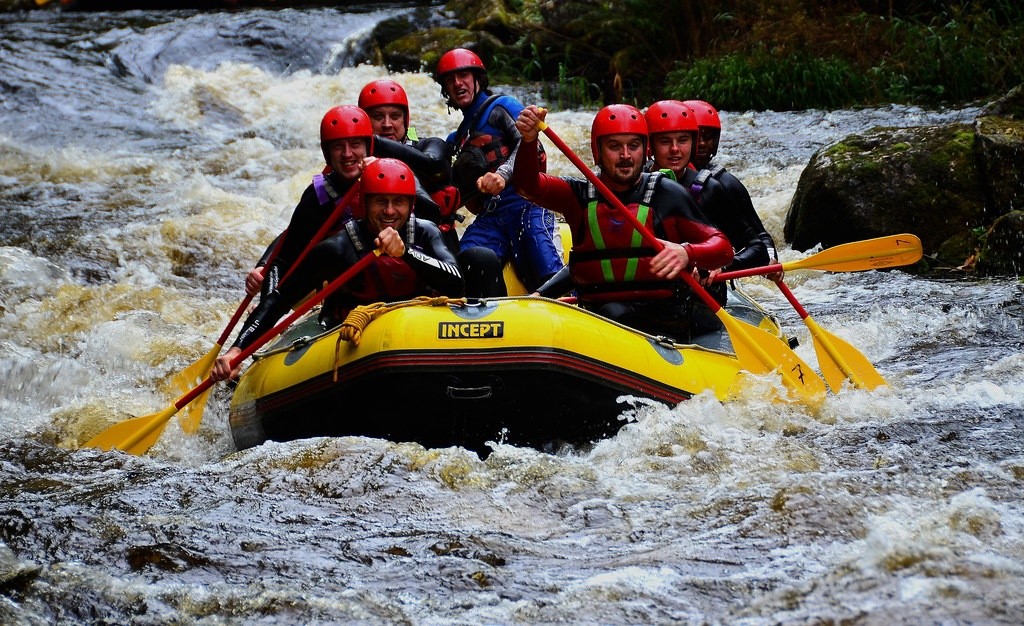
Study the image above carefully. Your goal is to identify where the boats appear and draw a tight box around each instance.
[229,274,790,459]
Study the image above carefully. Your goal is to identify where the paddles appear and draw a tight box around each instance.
[763,262,889,397]
[156,218,284,436]
[276,174,364,290]
[535,105,828,412]
[558,231,924,303]
[77,242,388,463]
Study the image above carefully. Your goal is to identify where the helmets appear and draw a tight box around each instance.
[591,104,649,166]
[683,101,721,157]
[358,80,410,130]
[320,105,374,166]
[436,48,489,90]
[644,100,699,161]
[361,158,416,221]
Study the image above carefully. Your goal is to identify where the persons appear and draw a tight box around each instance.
[210,49,784,381]
